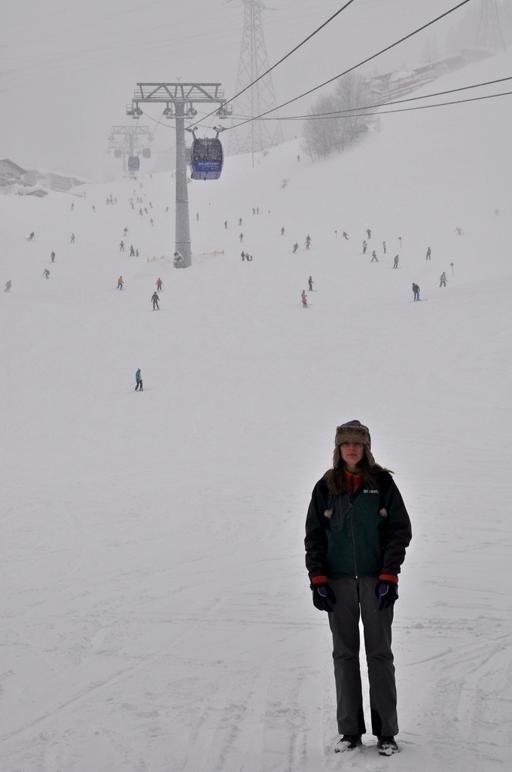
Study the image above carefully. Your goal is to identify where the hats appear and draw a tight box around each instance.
[332,419,376,468]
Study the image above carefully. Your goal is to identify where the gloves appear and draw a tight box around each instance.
[374,583,400,610]
[313,588,333,612]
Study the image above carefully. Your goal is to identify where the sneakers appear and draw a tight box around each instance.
[376,737,401,756]
[333,735,363,754]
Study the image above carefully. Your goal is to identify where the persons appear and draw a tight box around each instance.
[116,275,125,290]
[300,290,308,309]
[302,418,413,757]
[134,368,143,392]
[439,272,447,287]
[3,280,13,293]
[412,283,420,301]
[308,275,314,291]
[42,267,51,280]
[154,277,163,290]
[150,291,160,312]
